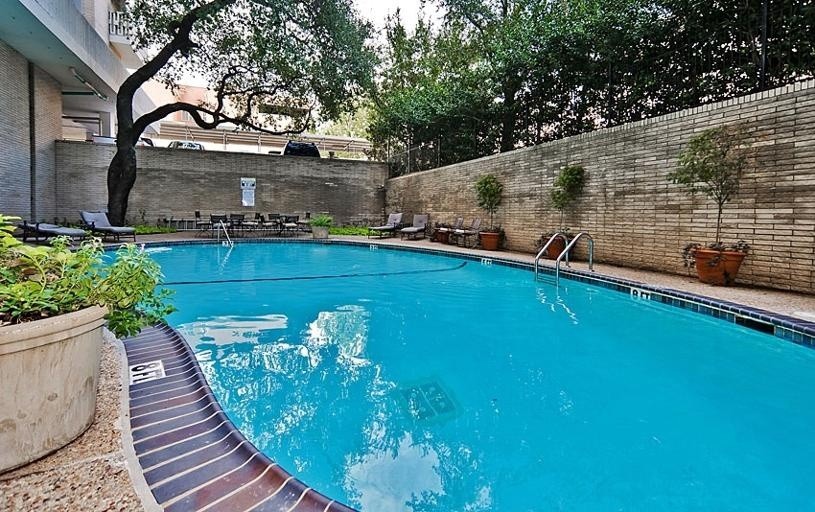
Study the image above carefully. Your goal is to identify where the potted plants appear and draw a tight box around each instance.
[309,212,332,238]
[542,166,590,260]
[665,119,769,285]
[471,174,505,250]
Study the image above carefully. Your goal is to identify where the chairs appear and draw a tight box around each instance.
[194,211,311,238]
[77,209,136,242]
[399,213,428,240]
[368,213,404,240]
[17,217,86,244]
[430,217,482,248]
